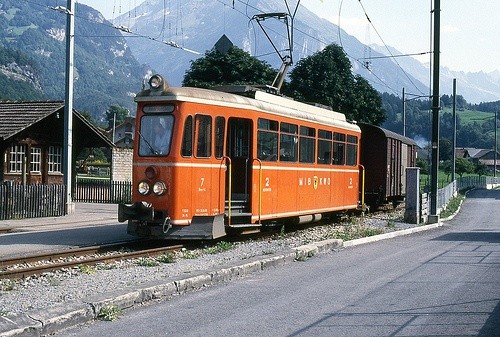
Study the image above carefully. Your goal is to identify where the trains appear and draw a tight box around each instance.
[117,74,417,245]
